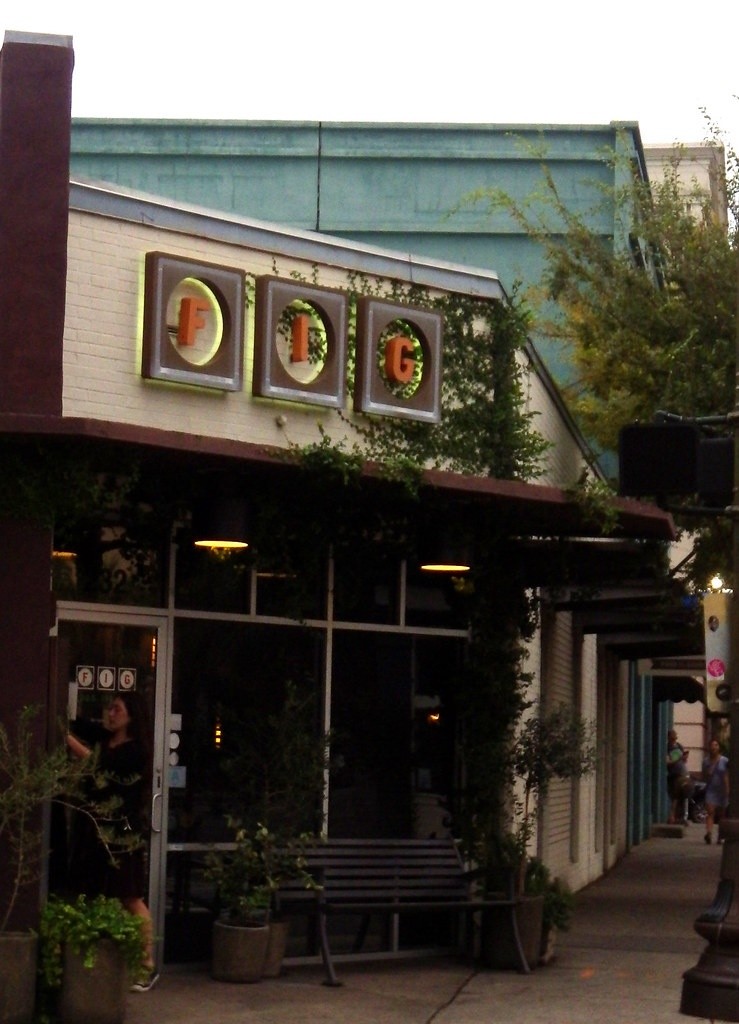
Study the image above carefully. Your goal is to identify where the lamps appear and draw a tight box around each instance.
[190,499,249,550]
[418,520,474,575]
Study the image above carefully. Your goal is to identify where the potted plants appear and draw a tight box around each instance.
[1,703,150,1023]
[467,697,626,973]
[202,815,328,986]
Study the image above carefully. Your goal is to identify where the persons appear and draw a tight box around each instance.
[666,730,696,823]
[56,692,159,992]
[701,739,730,845]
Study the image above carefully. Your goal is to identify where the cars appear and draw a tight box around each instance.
[679,777,711,823]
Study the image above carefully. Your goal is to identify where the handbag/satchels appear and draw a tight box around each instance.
[692,789,706,803]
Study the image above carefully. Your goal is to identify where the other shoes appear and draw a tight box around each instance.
[704,834,711,844]
[717,837,722,845]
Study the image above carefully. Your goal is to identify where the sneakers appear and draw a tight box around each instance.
[129,967,159,992]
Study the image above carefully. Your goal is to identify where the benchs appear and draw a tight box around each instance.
[288,837,530,987]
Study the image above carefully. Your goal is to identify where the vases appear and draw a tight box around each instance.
[267,919,291,979]
